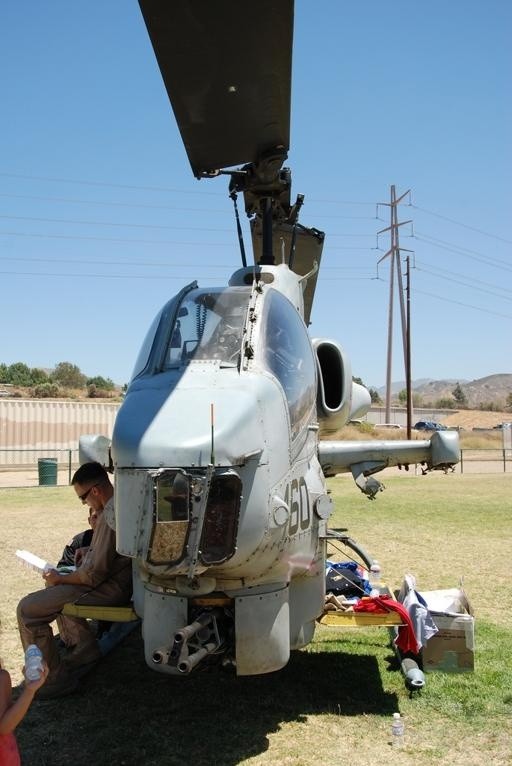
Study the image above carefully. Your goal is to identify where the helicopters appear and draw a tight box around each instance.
[79,2,470,691]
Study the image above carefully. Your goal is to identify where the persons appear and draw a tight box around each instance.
[17,462,134,696]
[0,660,50,766]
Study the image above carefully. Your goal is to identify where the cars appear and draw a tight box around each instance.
[306,420,511,434]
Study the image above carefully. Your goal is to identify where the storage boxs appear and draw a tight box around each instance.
[397,574,475,674]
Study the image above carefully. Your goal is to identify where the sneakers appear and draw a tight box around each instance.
[20,680,67,700]
[67,638,102,673]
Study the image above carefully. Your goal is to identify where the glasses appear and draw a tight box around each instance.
[79,484,98,501]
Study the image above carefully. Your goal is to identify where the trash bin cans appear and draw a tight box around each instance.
[38,458,57,485]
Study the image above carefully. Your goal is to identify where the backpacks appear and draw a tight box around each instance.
[325,569,365,598]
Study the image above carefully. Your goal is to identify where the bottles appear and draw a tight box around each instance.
[370,561,382,593]
[393,713,404,750]
[25,642,45,681]
